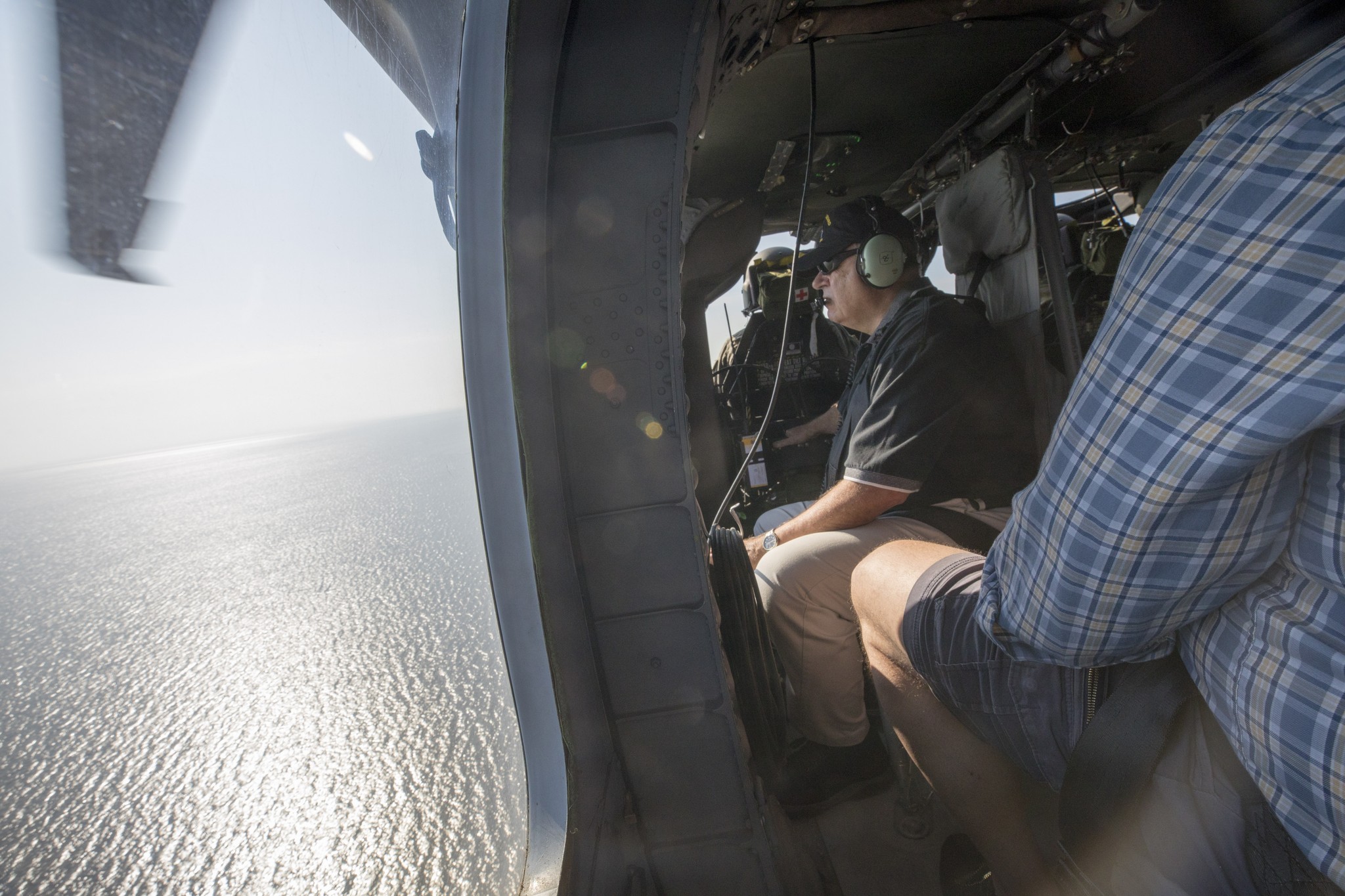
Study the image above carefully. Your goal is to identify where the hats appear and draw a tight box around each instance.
[796,194,915,268]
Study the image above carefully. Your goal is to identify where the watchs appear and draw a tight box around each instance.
[762,528,779,552]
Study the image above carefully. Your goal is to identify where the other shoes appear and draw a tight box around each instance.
[777,736,900,821]
[937,833,997,896]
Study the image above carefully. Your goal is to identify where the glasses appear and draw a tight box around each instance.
[815,248,858,276]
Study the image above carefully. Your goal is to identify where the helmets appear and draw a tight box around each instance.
[742,245,795,316]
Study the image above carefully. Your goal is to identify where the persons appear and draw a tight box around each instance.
[848,30,1345,896]
[706,195,1042,812]
[712,243,861,479]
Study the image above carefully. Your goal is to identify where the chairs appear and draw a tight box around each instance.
[737,266,851,422]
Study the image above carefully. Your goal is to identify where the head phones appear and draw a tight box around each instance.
[858,194,907,289]
